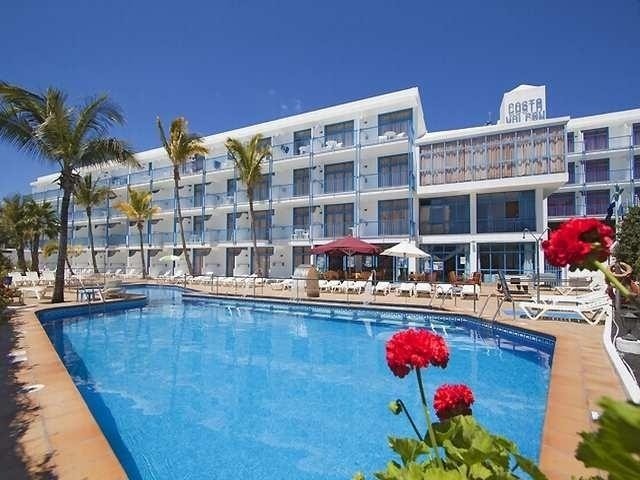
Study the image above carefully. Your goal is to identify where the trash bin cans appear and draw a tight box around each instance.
[4,277,12,285]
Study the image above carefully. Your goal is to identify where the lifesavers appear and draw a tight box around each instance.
[609,262,632,279]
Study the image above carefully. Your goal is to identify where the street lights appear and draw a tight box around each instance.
[523,227,553,305]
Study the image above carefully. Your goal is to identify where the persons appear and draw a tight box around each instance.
[407,272,416,281]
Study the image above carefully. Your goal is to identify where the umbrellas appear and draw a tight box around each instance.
[158,255,184,276]
[379,240,433,280]
[306,234,383,276]
